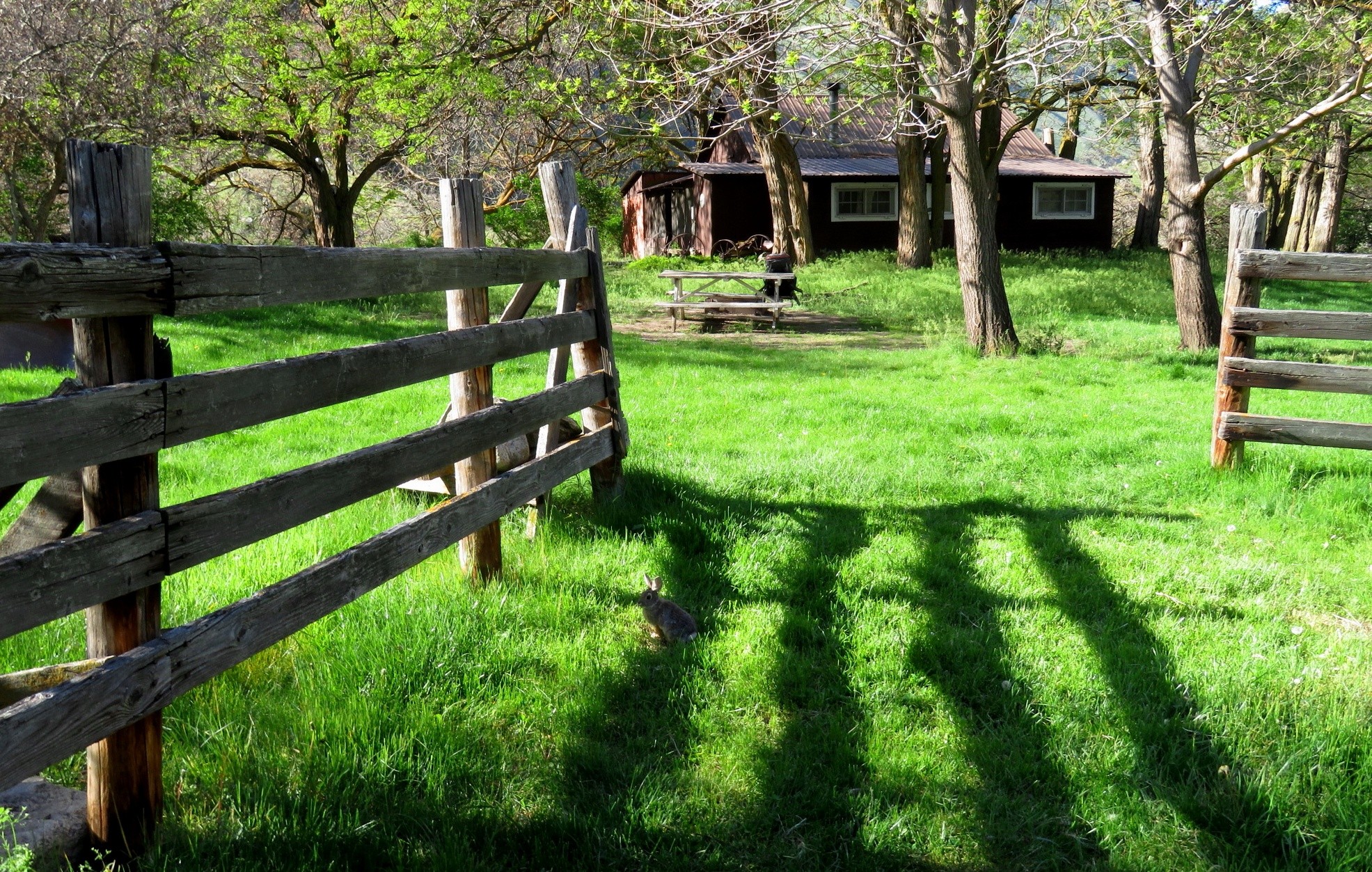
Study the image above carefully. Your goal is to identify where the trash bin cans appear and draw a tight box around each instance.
[764,252,798,297]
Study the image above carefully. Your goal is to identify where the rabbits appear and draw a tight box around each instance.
[638,572,698,645]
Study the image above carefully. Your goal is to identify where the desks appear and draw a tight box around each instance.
[657,269,796,333]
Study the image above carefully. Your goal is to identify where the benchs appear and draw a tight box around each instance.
[665,289,794,299]
[654,300,793,309]
